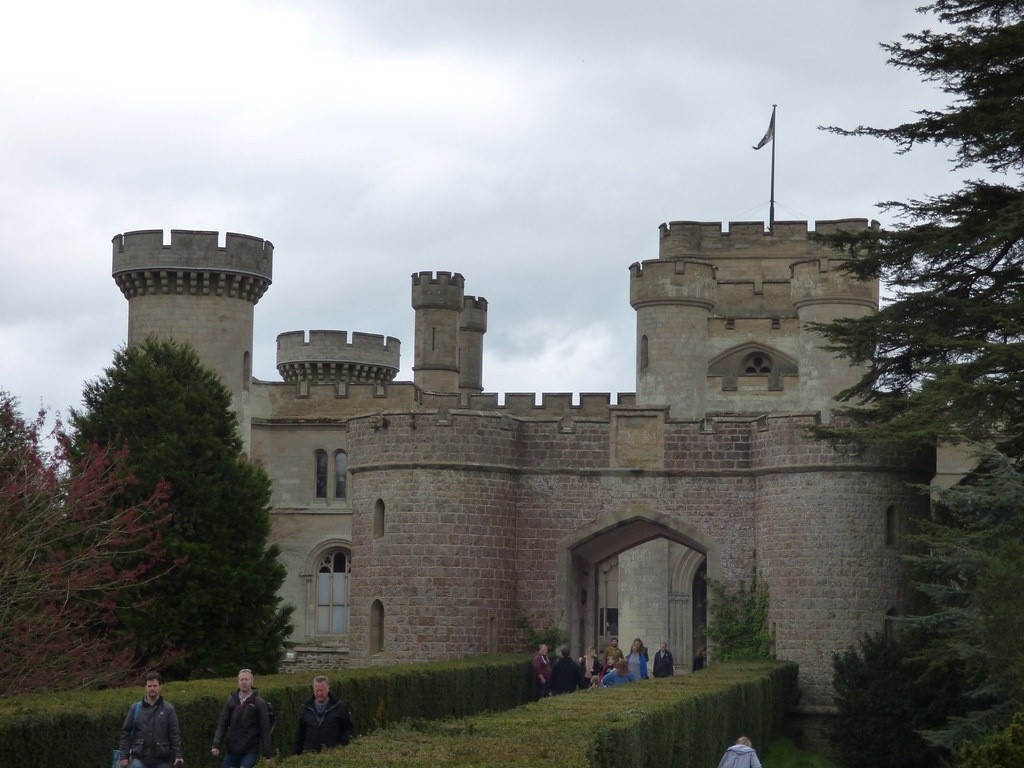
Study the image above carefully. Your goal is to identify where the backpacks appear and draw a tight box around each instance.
[229,695,276,738]
[550,660,574,691]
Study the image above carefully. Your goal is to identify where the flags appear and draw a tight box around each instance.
[753,112,774,150]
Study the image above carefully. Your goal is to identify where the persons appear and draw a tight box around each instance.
[718,736,762,768]
[211,669,272,768]
[692,649,706,671]
[293,676,351,755]
[533,638,674,701]
[119,673,186,768]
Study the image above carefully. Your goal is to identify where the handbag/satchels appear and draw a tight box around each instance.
[112,750,131,768]
